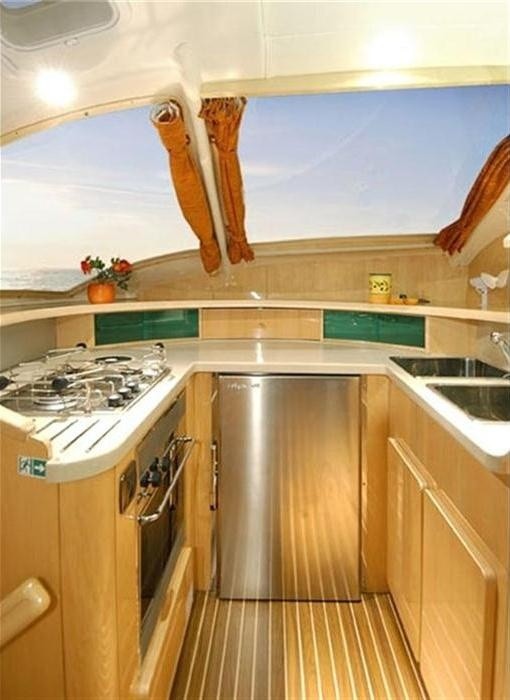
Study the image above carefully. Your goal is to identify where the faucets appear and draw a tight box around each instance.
[489,331,510,363]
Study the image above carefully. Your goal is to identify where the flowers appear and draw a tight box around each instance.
[81,256,133,292]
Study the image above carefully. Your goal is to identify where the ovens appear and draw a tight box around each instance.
[133,388,197,669]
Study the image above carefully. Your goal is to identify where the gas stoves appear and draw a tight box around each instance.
[0,340,170,417]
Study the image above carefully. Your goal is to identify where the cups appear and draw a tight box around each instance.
[368,272,391,303]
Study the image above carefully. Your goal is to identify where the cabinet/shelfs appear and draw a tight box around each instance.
[388,435,509,700]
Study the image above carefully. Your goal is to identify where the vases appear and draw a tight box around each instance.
[88,283,116,304]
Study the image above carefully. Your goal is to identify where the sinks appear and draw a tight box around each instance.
[389,356,510,377]
[427,385,510,423]
